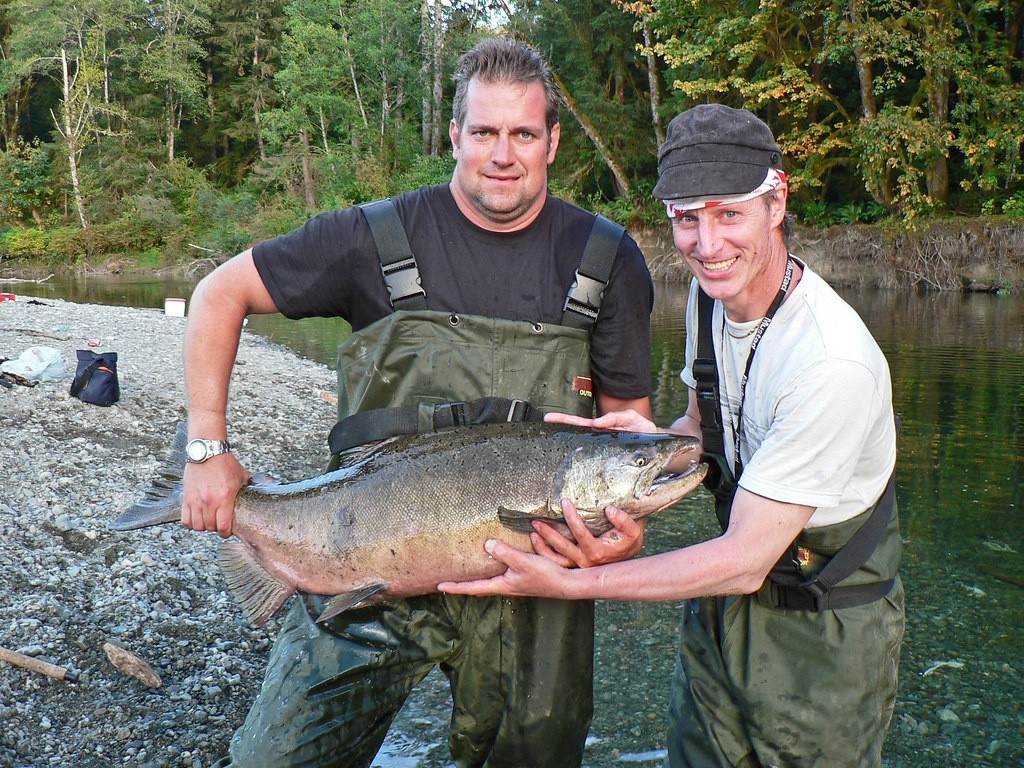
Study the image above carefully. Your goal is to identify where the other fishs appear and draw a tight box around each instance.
[110,420,708,627]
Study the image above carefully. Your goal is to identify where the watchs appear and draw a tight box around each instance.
[184,438,230,463]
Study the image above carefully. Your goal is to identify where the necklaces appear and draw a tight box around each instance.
[725,322,761,339]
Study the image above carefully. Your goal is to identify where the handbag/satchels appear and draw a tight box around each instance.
[69,350,121,407]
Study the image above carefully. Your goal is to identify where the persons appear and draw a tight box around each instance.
[433,101,907,768]
[178,34,655,768]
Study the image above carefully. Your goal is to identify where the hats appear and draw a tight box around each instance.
[652,104,785,199]
